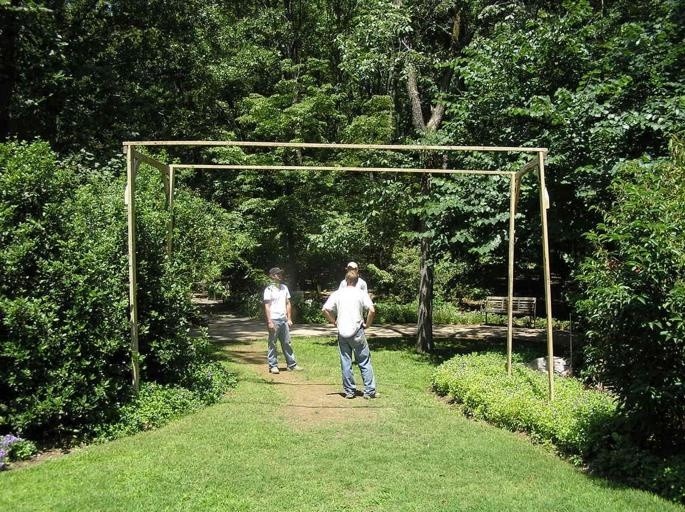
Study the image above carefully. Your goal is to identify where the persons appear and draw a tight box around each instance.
[338,262,368,366]
[263,267,303,374]
[321,270,381,399]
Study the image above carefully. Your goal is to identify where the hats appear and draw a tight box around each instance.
[348,262,357,268]
[269,267,283,276]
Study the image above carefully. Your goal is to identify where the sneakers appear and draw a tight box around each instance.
[287,365,303,371]
[269,367,279,374]
[364,393,380,399]
[346,393,354,399]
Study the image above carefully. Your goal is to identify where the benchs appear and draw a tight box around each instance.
[483,296,537,328]
